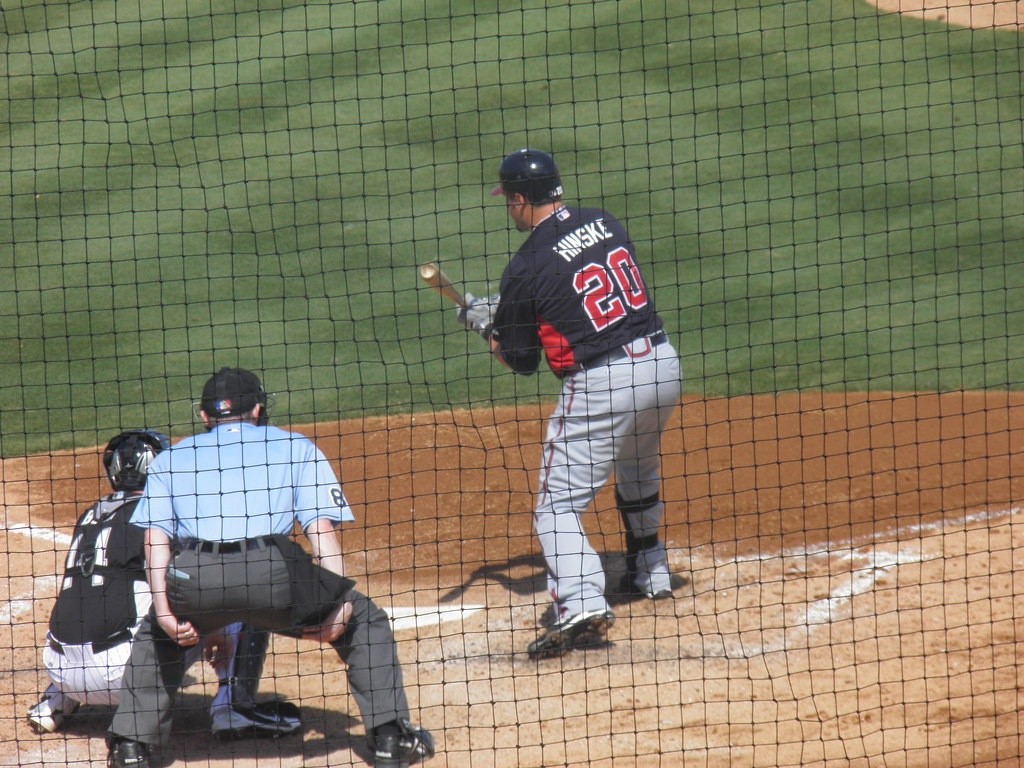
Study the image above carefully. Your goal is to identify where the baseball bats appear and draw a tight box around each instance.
[419,262,466,308]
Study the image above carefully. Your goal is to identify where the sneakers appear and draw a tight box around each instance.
[27,683,79,733]
[106,736,152,768]
[613,575,672,600]
[373,720,435,768]
[213,703,304,739]
[527,609,615,660]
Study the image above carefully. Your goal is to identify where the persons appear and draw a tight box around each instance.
[106,364,436,768]
[454,148,685,661]
[26,426,304,740]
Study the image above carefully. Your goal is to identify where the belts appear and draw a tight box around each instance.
[50,630,132,654]
[568,331,666,376]
[183,536,275,554]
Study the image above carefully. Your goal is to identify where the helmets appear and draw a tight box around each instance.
[200,367,267,417]
[489,151,564,205]
[103,431,164,492]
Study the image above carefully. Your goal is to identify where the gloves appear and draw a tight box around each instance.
[457,292,500,342]
